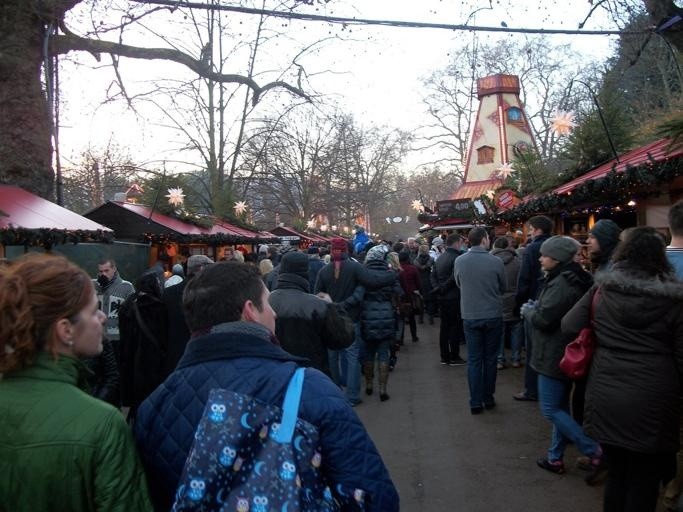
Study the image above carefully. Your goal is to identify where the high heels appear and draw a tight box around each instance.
[511,392,538,400]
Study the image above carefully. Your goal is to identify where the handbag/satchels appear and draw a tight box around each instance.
[558,327,595,380]
[171,367,371,511]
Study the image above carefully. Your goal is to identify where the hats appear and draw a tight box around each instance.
[187,254,215,268]
[280,251,309,276]
[539,219,623,262]
[331,225,390,262]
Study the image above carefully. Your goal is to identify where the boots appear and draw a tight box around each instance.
[363,339,399,401]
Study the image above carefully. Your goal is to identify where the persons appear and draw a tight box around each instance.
[434,229,528,414]
[0,248,153,511]
[514,216,617,473]
[657,200,683,282]
[314,226,437,406]
[78,338,118,398]
[88,256,134,339]
[560,226,683,512]
[132,261,403,512]
[120,242,356,420]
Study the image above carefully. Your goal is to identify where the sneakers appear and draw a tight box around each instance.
[497,361,520,369]
[471,397,496,415]
[440,359,467,365]
[537,446,606,474]
